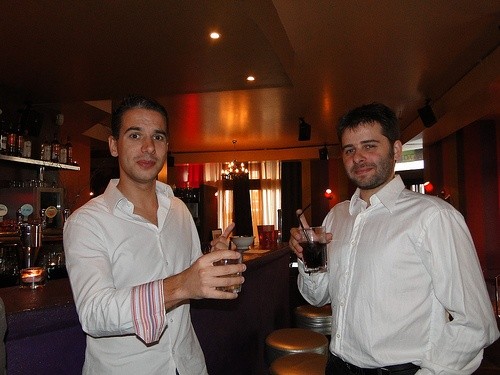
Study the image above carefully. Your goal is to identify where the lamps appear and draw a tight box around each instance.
[419,99,437,127]
[319,145,329,161]
[423,181,433,193]
[223,160,248,189]
[324,188,334,199]
[298,116,311,141]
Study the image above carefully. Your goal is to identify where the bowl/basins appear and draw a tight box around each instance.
[230,236,255,249]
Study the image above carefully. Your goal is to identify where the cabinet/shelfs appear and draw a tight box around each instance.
[0,154,81,246]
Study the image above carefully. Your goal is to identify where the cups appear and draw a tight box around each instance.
[296,226,327,273]
[18,246,47,288]
[0,243,66,285]
[274,230,279,249]
[212,250,243,293]
[257,225,274,250]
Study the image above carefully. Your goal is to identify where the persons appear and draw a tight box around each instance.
[62,96,246,375]
[290,102,500,375]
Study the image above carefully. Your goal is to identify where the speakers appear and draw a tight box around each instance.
[319,147,329,160]
[417,104,438,128]
[297,121,312,141]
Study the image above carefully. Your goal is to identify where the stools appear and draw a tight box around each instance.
[268,352,328,375]
[293,304,332,335]
[265,327,329,356]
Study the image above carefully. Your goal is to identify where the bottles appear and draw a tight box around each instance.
[16,121,32,158]
[65,136,74,165]
[51,136,60,163]
[60,139,67,163]
[53,111,68,146]
[0,121,16,156]
[40,136,51,161]
